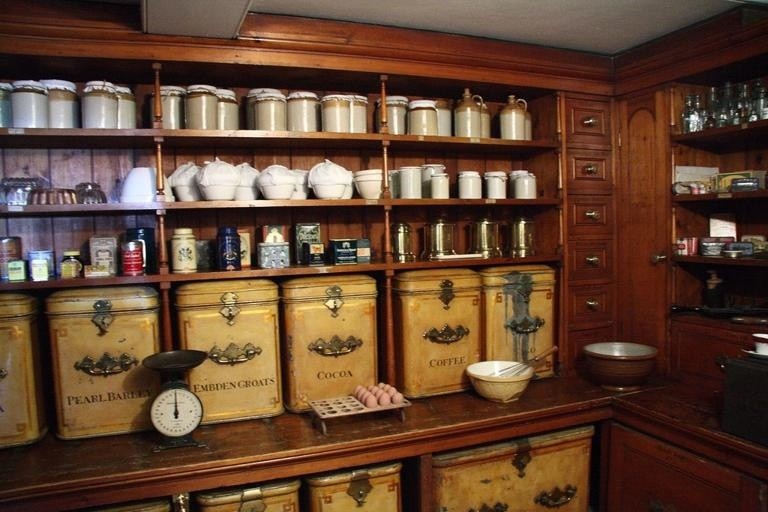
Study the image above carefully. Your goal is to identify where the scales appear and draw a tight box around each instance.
[142,350,208,453]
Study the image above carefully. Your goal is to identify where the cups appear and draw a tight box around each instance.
[751,333,768,356]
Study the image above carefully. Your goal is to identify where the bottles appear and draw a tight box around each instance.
[700,269,723,309]
[215,226,240,271]
[679,77,768,135]
[171,228,195,272]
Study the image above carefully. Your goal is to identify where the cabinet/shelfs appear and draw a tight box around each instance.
[607,377,767,511]
[377,24,563,411]
[0,0,170,446]
[561,90,613,362]
[666,1,768,399]
[154,11,389,431]
[612,85,677,380]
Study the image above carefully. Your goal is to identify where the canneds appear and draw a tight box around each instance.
[120,240,143,276]
[60,251,84,278]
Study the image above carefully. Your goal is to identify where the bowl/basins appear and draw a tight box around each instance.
[582,340,660,391]
[120,167,174,203]
[171,168,396,202]
[464,359,535,405]
[75,183,106,205]
[27,188,78,206]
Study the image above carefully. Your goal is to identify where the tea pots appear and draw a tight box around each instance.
[452,87,533,142]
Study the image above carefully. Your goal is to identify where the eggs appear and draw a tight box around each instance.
[353,383,403,408]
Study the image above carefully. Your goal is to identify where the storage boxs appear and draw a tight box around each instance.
[43,284,165,442]
[1,290,50,446]
[196,478,302,510]
[392,267,480,401]
[305,461,405,510]
[432,422,596,511]
[479,266,558,383]
[172,278,285,428]
[280,274,378,416]
